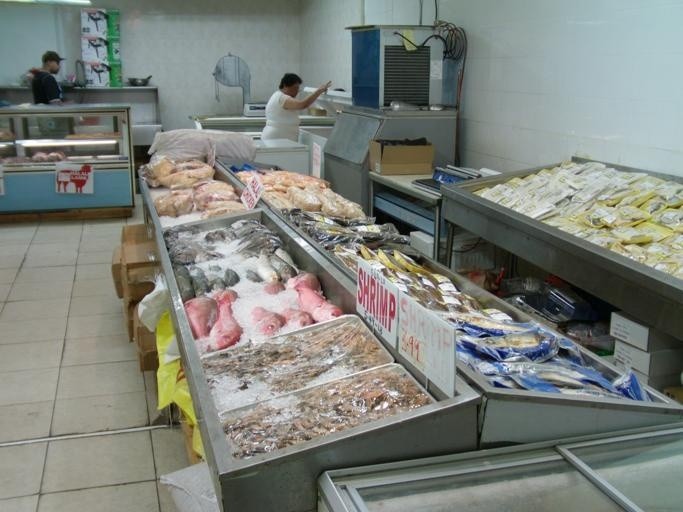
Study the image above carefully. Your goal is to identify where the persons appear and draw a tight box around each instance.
[260,71,333,141]
[30,50,74,156]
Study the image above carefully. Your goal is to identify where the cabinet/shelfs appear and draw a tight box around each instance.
[138,157,682,512]
[0,103,136,216]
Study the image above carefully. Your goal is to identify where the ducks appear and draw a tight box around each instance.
[158,161,215,189]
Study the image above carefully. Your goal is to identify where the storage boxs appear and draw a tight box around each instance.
[111,223,204,465]
[410,231,495,272]
[369,140,435,175]
[610,311,683,393]
[81,7,122,87]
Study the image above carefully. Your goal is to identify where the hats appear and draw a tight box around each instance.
[42,51,65,62]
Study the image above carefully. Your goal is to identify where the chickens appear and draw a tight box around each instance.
[153,180,248,220]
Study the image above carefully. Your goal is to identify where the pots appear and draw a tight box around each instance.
[127,75,152,86]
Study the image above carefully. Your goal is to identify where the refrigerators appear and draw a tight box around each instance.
[295,84,356,180]
[256,137,313,177]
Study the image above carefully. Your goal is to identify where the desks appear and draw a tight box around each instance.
[369,172,442,261]
